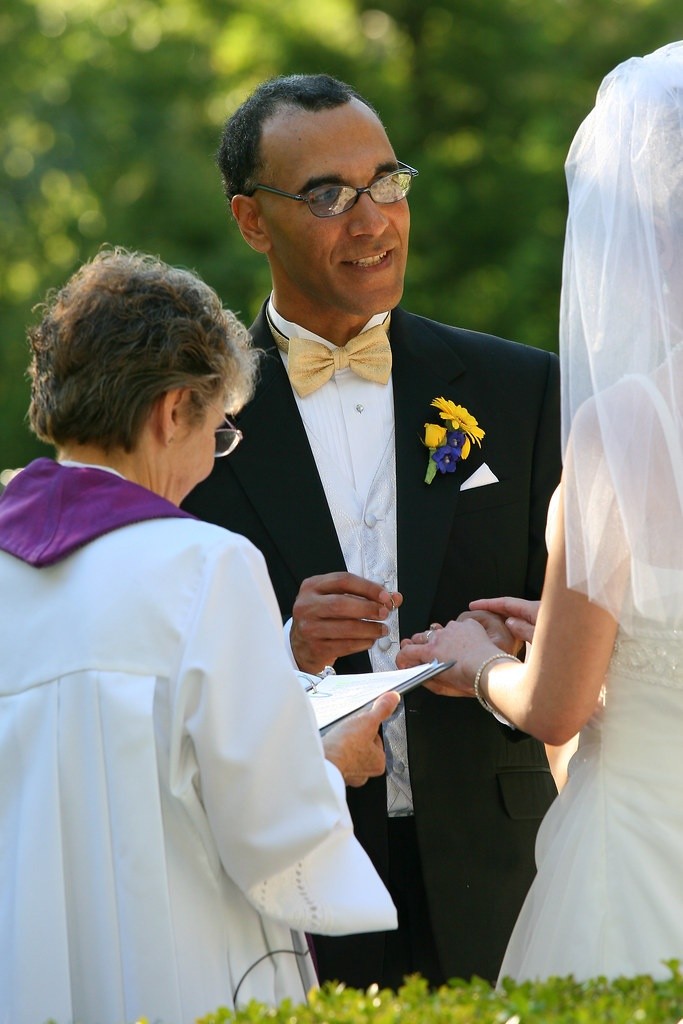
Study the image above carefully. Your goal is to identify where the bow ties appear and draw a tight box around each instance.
[264,302,392,398]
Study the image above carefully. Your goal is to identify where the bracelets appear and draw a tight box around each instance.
[473,653,523,716]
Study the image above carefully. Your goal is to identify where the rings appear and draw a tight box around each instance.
[427,630,432,639]
[383,591,396,612]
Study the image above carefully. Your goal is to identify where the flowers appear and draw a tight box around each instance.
[419,397,486,484]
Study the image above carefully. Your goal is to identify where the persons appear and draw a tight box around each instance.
[399,30,683,982]
[180,68,615,993]
[0,246,406,1022]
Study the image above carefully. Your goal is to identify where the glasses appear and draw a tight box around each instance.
[247,159,419,218]
[190,384,243,457]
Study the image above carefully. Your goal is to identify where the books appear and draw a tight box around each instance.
[295,658,459,742]
